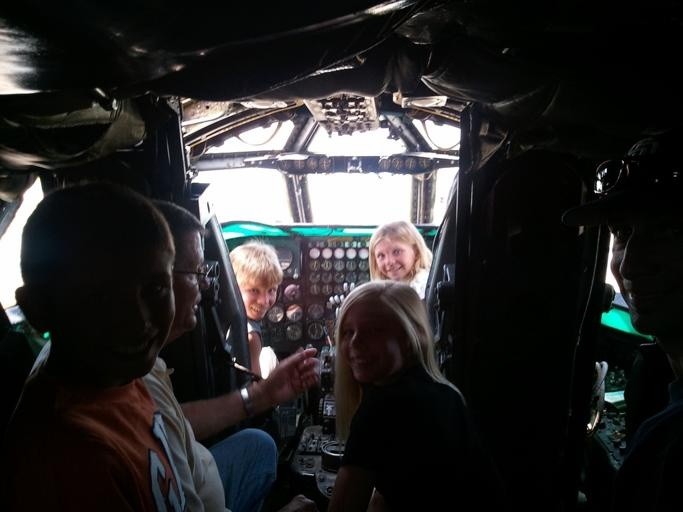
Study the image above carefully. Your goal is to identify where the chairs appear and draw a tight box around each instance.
[425,123,616,380]
[192,182,253,398]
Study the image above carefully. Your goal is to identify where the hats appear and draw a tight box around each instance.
[561,136,683,228]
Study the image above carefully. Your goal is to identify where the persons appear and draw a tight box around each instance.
[363,220,433,302]
[144,197,320,512]
[9,184,200,512]
[225,241,279,382]
[329,278,464,512]
[562,133,683,512]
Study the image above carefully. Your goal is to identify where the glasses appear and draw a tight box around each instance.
[172,264,215,285]
[594,156,682,195]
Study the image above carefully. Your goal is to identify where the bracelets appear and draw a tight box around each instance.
[240,388,255,421]
